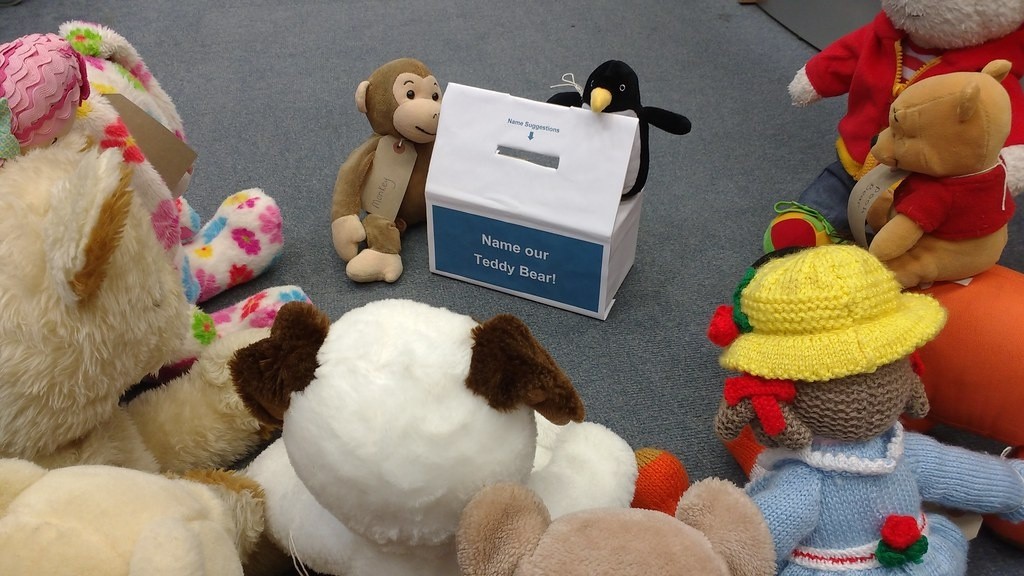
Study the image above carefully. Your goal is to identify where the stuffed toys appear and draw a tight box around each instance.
[545,59,691,200]
[0,0,1024,576]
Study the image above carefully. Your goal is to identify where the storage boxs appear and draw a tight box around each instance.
[421,78,647,323]
